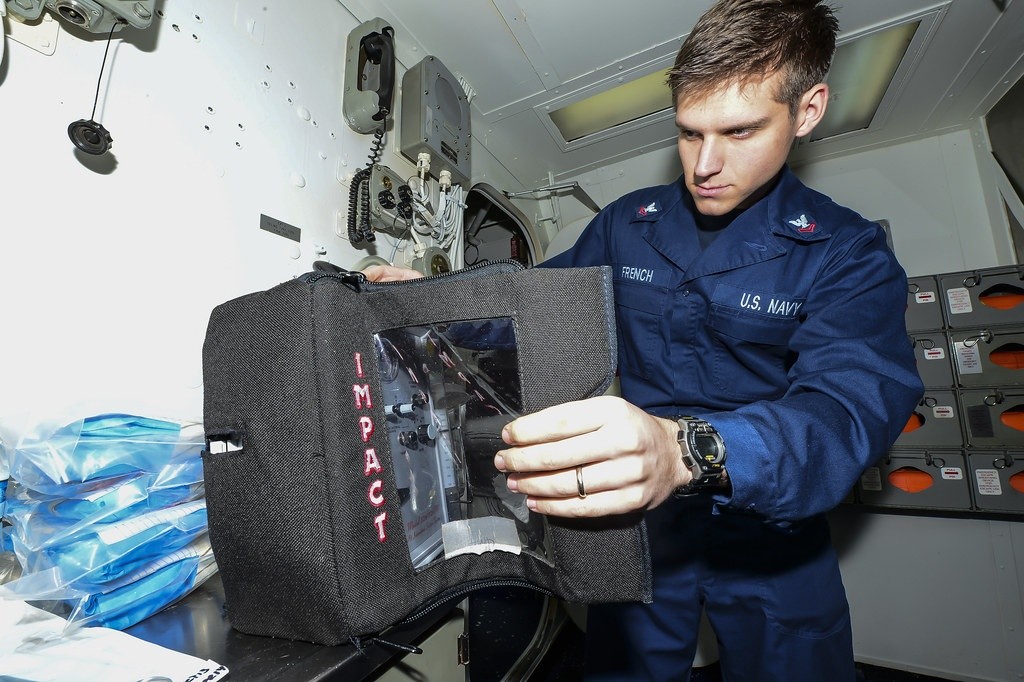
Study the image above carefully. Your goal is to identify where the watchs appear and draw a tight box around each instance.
[663,412,728,497]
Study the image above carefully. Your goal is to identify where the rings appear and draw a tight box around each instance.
[574,465,586,498]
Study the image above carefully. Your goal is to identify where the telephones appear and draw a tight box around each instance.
[344,15,397,133]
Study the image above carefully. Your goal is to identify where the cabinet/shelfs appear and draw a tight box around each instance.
[852,264,1024,516]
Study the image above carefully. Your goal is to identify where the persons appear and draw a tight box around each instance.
[360,0,926,682]
